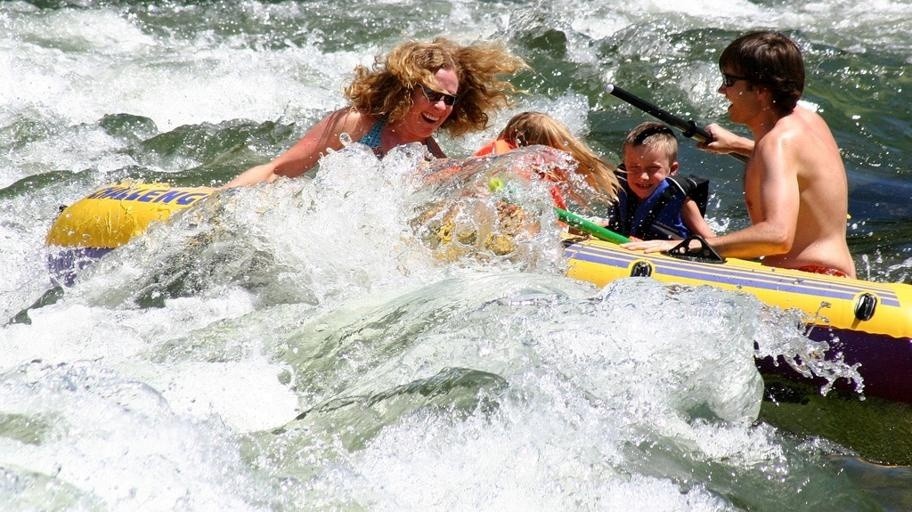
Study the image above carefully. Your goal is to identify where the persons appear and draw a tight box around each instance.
[219,38,533,194]
[607,122,716,242]
[618,30,857,281]
[416,111,625,243]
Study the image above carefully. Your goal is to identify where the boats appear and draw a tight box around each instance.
[45,184,912,399]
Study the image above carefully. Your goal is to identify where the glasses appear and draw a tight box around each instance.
[721,73,746,88]
[415,82,456,106]
